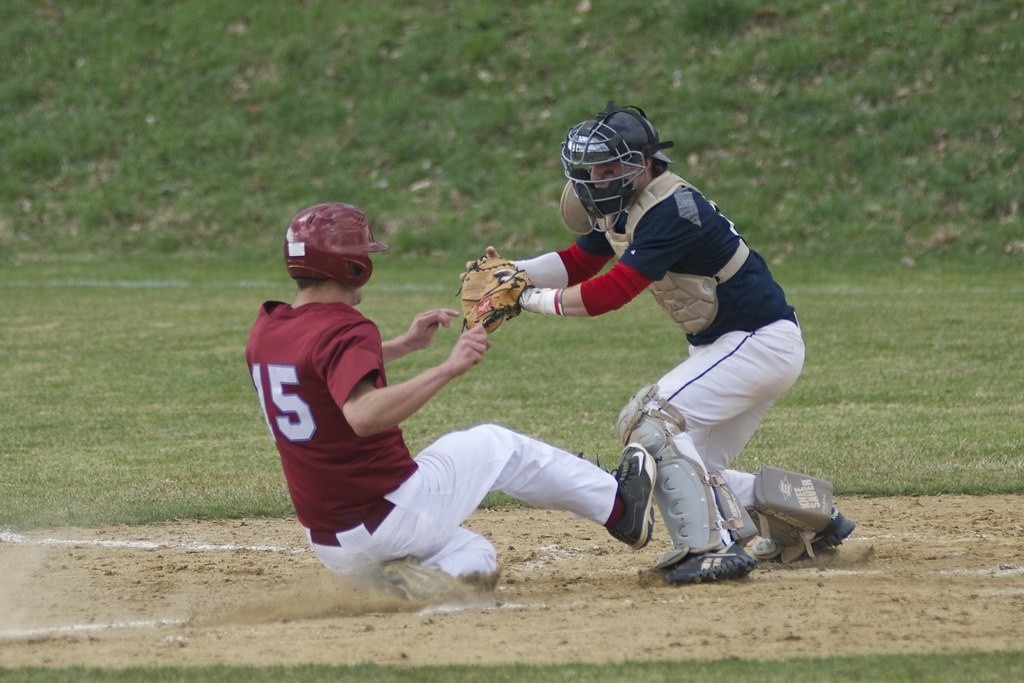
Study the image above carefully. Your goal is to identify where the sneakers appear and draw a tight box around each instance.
[666,542,758,585]
[776,507,855,562]
[385,556,458,599]
[597,443,657,549]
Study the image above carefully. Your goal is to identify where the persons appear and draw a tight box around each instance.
[459,99,855,585]
[244,200,658,597]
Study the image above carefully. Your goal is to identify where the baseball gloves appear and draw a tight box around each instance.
[453,255,536,335]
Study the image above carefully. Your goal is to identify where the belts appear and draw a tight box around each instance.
[782,312,797,324]
[311,500,395,546]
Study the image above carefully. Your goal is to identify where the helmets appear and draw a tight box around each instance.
[285,202,388,289]
[595,107,675,164]
